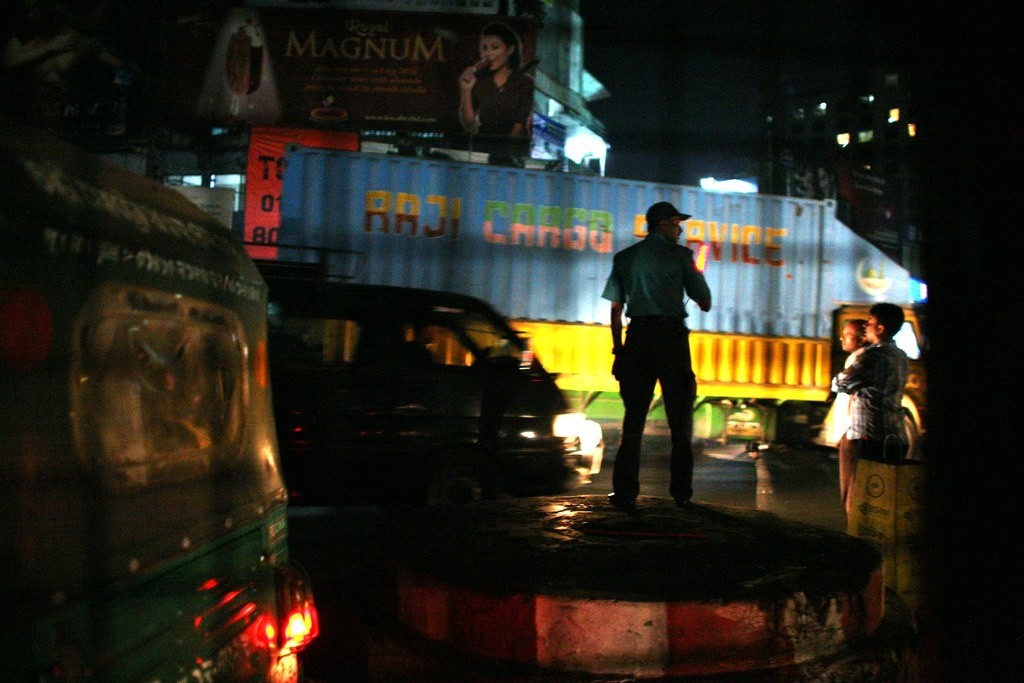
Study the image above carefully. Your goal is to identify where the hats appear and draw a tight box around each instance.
[645,201,692,223]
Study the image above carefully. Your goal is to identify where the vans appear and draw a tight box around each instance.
[244,241,606,523]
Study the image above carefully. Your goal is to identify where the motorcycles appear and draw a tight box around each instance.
[0,121,318,683]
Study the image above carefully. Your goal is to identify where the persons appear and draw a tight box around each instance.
[606,201,712,510]
[411,322,471,372]
[456,22,532,139]
[830,302,911,537]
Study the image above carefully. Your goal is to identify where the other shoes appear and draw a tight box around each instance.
[674,497,689,506]
[606,492,637,509]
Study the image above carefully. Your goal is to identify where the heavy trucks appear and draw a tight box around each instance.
[268,144,928,471]
